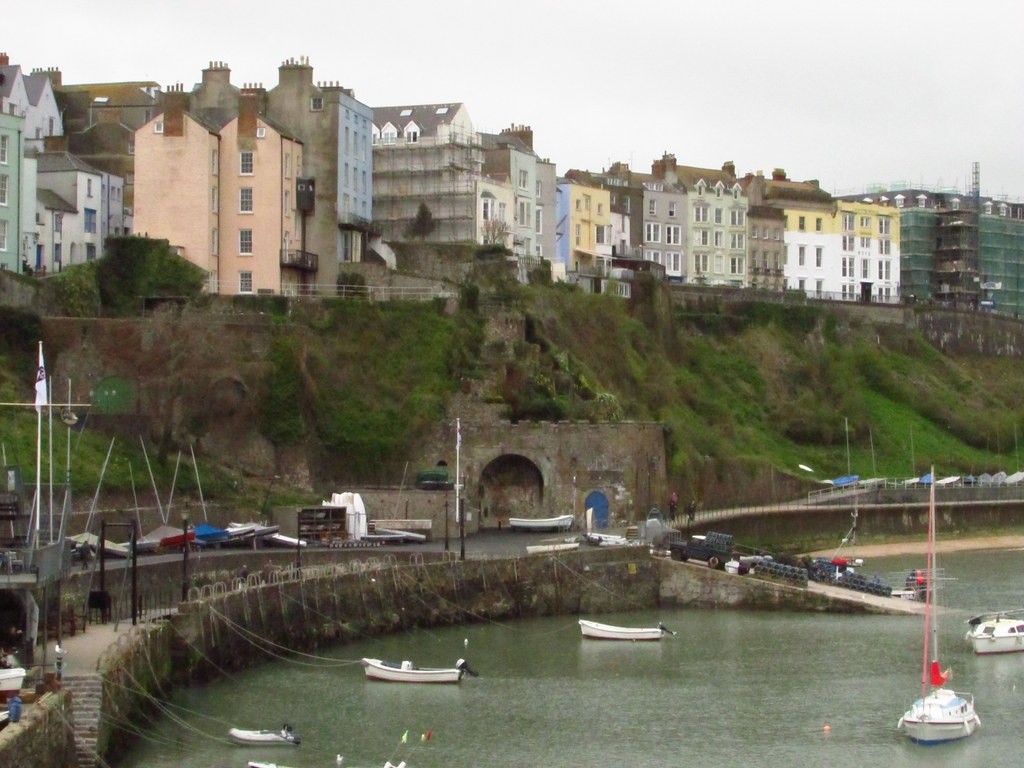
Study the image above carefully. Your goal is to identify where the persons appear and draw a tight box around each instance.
[238,565,249,590]
[667,491,697,520]
[738,561,748,576]
[8,694,23,723]
[79,540,90,568]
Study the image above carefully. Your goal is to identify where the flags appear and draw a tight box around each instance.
[34,345,47,413]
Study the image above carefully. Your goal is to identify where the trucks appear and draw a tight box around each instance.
[669,532,731,569]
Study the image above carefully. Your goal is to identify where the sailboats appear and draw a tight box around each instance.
[858,426,888,487]
[833,414,859,488]
[896,464,981,746]
[905,425,920,484]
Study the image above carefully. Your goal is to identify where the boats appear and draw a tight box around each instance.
[362,658,479,682]
[228,521,307,547]
[508,515,574,530]
[965,618,1024,654]
[116,521,279,551]
[578,619,677,639]
[228,724,301,747]
[375,527,426,542]
[0,668,26,698]
[582,531,628,546]
[526,542,579,554]
